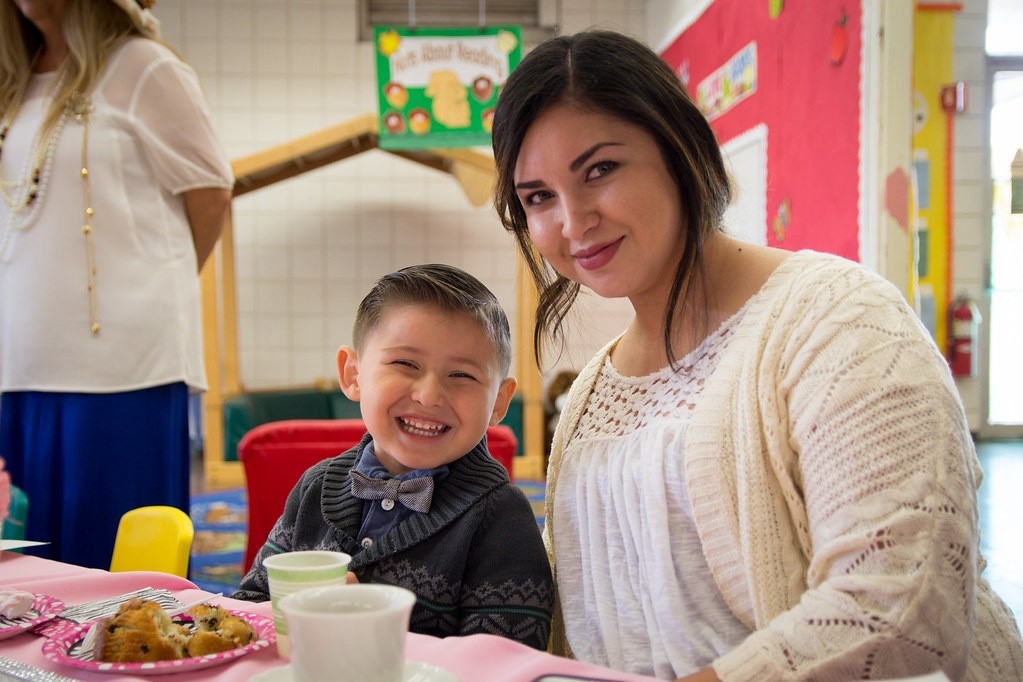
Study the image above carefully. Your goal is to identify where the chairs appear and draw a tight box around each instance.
[109,506,194,579]
[0,484,27,554]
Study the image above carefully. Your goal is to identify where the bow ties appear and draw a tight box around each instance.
[349,470,434,514]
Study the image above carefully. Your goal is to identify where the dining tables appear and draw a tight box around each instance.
[0,537,664,682]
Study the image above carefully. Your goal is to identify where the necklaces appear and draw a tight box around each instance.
[1,46,73,255]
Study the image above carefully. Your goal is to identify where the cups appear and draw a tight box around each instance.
[281,582,417,682]
[263,550,352,657]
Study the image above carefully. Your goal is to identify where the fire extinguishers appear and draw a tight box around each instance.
[945,293,983,379]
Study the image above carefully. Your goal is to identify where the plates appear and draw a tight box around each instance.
[41,609,276,675]
[0,592,67,641]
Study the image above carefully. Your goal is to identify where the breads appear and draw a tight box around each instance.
[185,602,258,657]
[102,599,192,662]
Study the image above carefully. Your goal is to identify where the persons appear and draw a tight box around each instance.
[495,28,1022,681]
[0,0,236,583]
[224,262,556,653]
